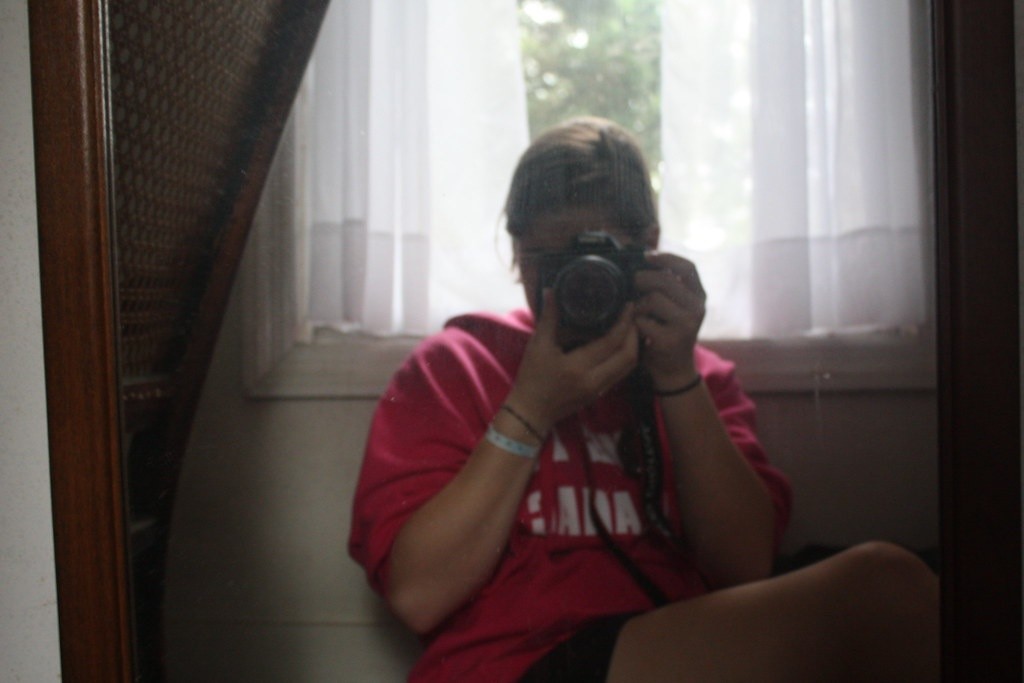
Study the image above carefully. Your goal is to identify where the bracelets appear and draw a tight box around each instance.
[654,374,703,398]
[484,424,542,460]
[500,403,547,447]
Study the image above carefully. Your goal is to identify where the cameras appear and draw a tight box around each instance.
[538,231,664,336]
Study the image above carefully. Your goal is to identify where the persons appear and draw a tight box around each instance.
[346,117,945,683]
